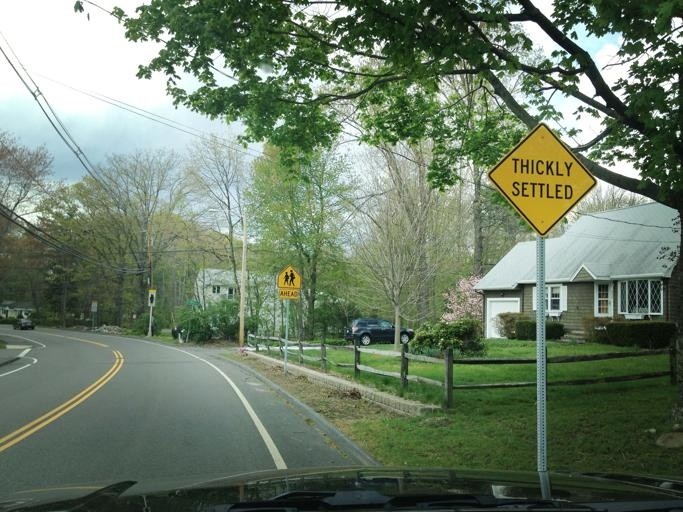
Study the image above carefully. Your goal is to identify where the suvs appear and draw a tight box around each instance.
[345,317,414,345]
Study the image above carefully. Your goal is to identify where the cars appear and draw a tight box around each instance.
[14,318,36,330]
